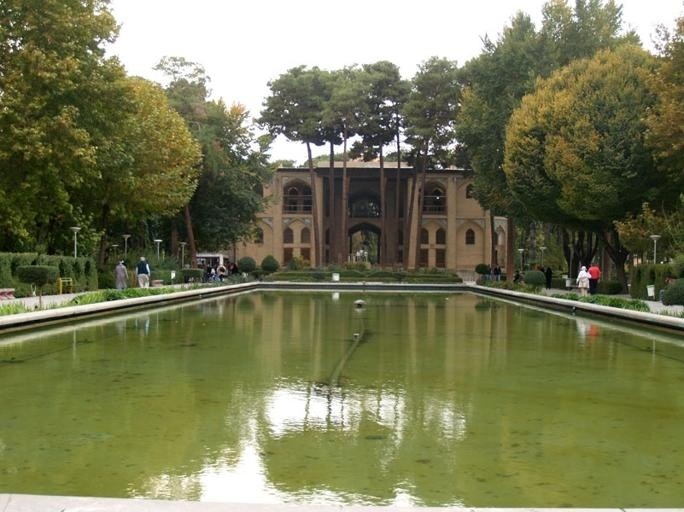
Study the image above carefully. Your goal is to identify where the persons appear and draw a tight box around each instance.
[576,266,592,297]
[513,270,520,281]
[546,267,552,289]
[198,258,239,277]
[136,257,150,287]
[115,258,129,289]
[535,265,545,272]
[587,263,601,295]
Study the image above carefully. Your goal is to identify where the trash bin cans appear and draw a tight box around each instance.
[59,278,72,295]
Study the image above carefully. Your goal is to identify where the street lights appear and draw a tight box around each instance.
[517,248,525,272]
[111,244,118,260]
[69,226,81,259]
[179,241,187,269]
[539,247,546,269]
[122,234,131,258]
[153,239,163,261]
[649,234,661,265]
